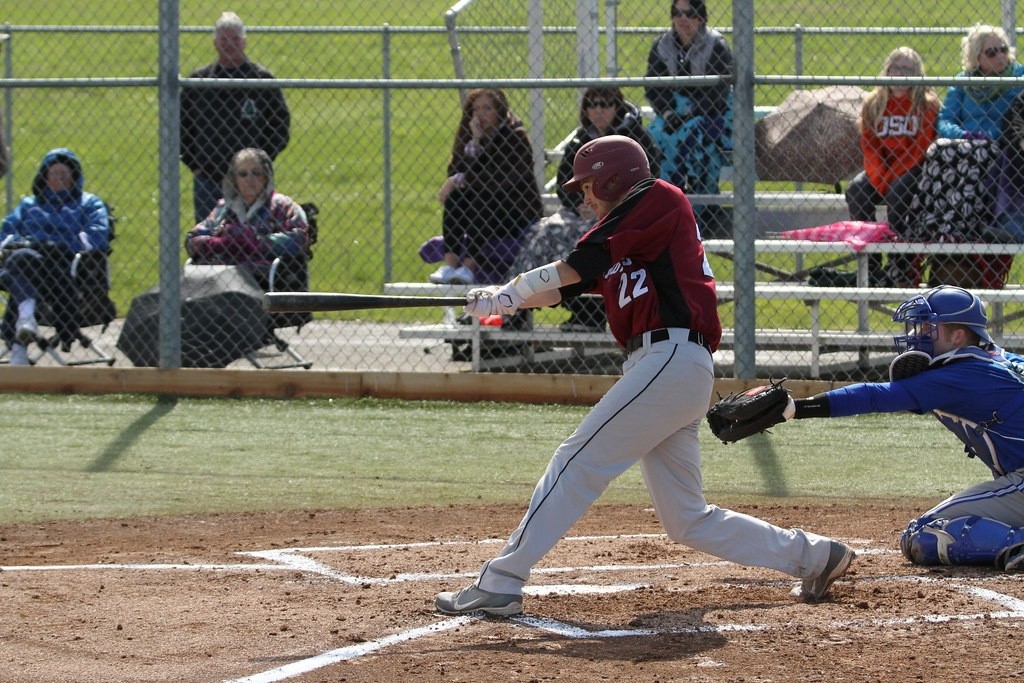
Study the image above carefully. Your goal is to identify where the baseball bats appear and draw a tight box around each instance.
[261,290,468,314]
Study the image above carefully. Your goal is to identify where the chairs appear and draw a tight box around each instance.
[185,202,321,370]
[0,203,118,367]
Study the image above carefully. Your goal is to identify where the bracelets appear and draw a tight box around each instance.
[471,135,480,139]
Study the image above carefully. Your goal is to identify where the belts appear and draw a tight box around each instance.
[622,328,713,361]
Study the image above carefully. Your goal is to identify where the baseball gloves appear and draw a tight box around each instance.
[705,378,797,445]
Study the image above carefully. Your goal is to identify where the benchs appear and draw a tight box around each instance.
[383,101,1023,378]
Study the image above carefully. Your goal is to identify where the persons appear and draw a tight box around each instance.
[184,148,309,293]
[556,87,661,333]
[706,285,1024,572]
[180,12,290,225]
[434,135,855,617]
[428,88,544,285]
[644,0,733,233]
[0,148,111,364]
[845,22,1024,290]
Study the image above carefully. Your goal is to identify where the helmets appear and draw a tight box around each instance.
[905,284,994,344]
[562,135,650,201]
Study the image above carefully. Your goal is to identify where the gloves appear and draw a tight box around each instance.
[463,273,535,319]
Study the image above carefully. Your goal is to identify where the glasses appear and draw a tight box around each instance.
[586,99,616,108]
[980,44,1009,58]
[238,172,260,177]
[888,64,914,75]
[672,7,698,19]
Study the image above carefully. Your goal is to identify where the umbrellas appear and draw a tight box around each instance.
[115,264,280,368]
[755,85,870,185]
[765,221,898,252]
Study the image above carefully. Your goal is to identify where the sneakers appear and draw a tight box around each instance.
[10,343,30,366]
[789,540,856,598]
[435,584,523,617]
[15,314,37,346]
[429,265,475,285]
